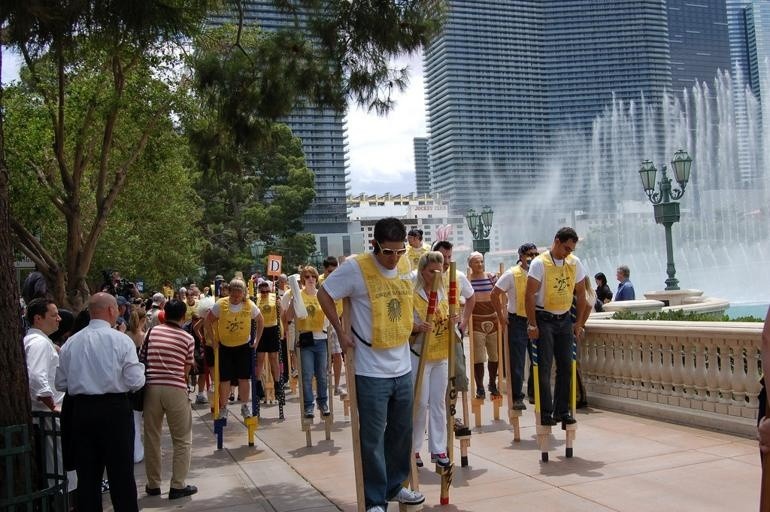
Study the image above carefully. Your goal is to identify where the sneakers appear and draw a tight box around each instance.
[415,452,423,467]
[101,479,110,494]
[168,484,198,500]
[541,415,556,426]
[553,413,577,424]
[488,385,499,395]
[476,388,485,398]
[513,399,526,410]
[528,397,535,404]
[430,451,450,468]
[453,418,469,433]
[366,505,386,512]
[389,486,426,505]
[194,367,348,423]
[145,484,161,496]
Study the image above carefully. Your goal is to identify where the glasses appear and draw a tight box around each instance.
[525,252,539,258]
[376,240,406,257]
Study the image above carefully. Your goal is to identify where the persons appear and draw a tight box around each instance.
[595,272,611,303]
[614,264,635,301]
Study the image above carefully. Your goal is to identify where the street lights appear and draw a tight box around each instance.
[309,250,324,275]
[199,266,208,289]
[637,149,693,289]
[248,236,267,275]
[464,204,495,274]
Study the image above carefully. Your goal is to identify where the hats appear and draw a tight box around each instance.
[215,275,225,281]
[152,292,166,307]
[117,296,131,306]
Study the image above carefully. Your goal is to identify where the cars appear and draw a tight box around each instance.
[136,281,143,292]
